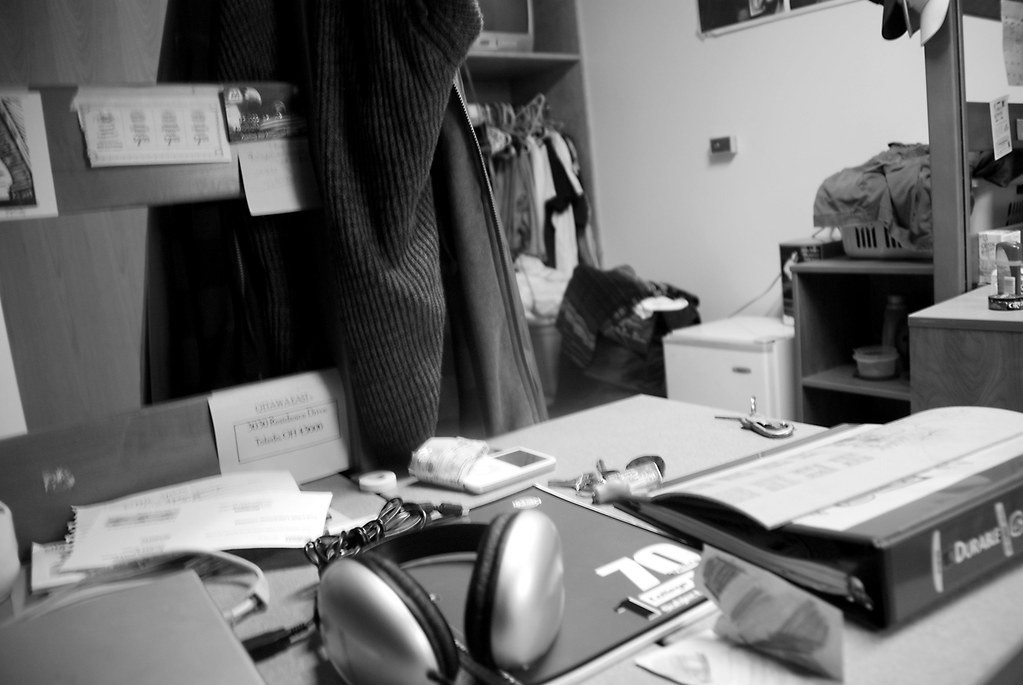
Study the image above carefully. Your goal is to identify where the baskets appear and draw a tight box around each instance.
[837,216,933,258]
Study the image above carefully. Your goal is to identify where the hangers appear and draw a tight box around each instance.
[474,93,560,161]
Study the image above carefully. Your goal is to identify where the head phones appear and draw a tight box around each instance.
[313,507,564,685]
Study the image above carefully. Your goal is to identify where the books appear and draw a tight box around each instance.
[613,406,1023,630]
[397,484,720,684]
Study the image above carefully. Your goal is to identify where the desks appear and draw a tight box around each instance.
[0,387,1023,685]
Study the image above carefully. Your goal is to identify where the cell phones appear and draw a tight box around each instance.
[462,445,558,494]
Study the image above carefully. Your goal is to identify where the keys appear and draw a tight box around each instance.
[549,472,596,489]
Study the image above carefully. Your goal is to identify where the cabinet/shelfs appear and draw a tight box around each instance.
[793,0,1022,426]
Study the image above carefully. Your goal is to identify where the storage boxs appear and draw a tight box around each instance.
[822,176,935,257]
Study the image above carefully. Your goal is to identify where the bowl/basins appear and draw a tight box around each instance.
[853,346,899,379]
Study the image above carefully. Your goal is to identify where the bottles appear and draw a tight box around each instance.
[883,295,908,370]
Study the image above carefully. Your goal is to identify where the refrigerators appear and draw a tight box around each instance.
[662,315,796,423]
